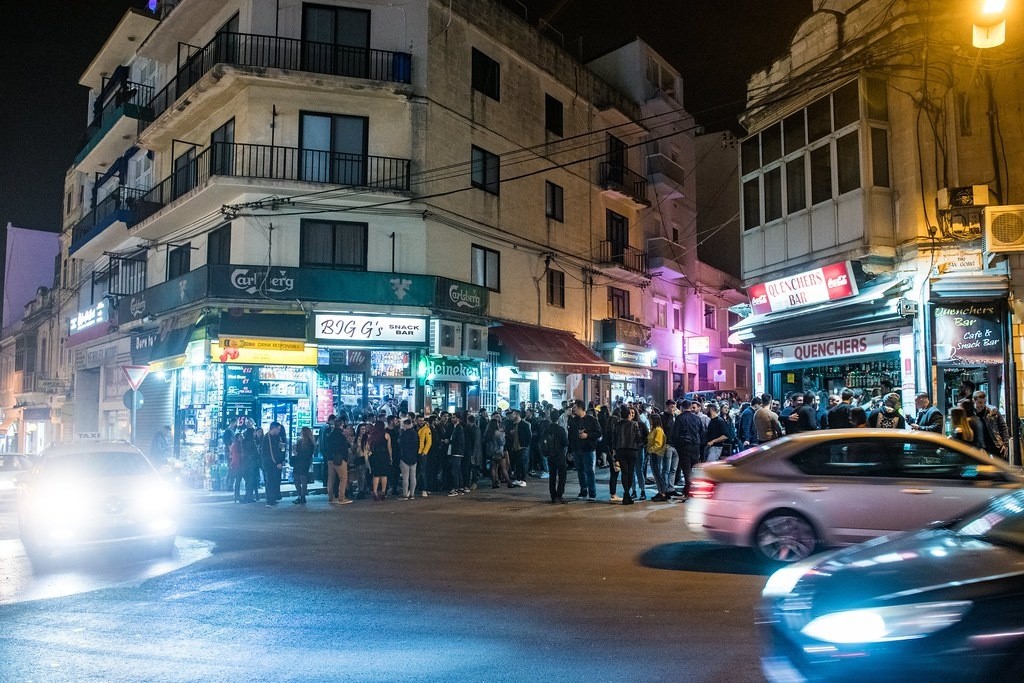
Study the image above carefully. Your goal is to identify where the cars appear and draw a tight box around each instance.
[0,453,33,540]
[755,488,1024,683]
[20,431,177,567]
[687,429,1024,567]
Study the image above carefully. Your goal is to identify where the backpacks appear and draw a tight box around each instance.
[540,431,555,457]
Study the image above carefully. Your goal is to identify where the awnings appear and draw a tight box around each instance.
[728,278,909,331]
[488,319,653,379]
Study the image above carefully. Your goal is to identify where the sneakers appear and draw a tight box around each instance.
[631,494,638,500]
[589,493,596,500]
[512,479,526,487]
[577,490,588,498]
[396,496,414,501]
[540,472,549,478]
[328,498,354,504]
[638,495,647,502]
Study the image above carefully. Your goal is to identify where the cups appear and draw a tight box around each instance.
[579,429,583,434]
[280,443,285,451]
[934,344,956,360]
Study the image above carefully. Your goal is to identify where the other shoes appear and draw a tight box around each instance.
[375,495,386,501]
[645,479,655,485]
[622,496,634,505]
[552,497,568,504]
[651,491,692,503]
[292,498,306,504]
[609,494,623,503]
[419,491,431,497]
[265,502,280,509]
[448,488,470,496]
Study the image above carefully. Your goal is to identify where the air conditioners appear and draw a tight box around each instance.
[429,319,462,356]
[981,205,1024,255]
[462,324,488,358]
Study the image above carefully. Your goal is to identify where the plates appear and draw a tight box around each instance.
[932,359,959,363]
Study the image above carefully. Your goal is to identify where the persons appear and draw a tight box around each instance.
[151,384,1009,510]
[5,455,23,471]
